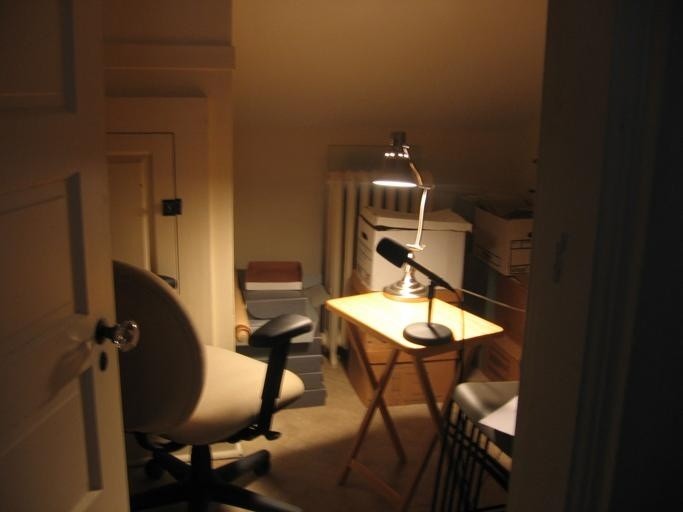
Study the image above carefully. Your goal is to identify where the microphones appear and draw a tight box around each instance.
[375,237,457,294]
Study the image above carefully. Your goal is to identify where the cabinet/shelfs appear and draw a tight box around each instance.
[94,0,243,465]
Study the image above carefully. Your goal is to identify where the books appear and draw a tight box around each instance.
[244,260,302,291]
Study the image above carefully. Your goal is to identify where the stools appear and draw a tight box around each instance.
[232,262,326,408]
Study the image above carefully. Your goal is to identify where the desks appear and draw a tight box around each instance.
[323,286,508,512]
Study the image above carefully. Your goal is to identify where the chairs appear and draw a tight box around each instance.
[110,256,308,512]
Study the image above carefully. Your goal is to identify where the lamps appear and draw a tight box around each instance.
[372,128,435,304]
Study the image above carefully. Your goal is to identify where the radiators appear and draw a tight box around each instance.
[325,168,443,367]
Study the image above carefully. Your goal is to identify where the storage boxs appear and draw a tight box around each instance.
[347,195,536,408]
